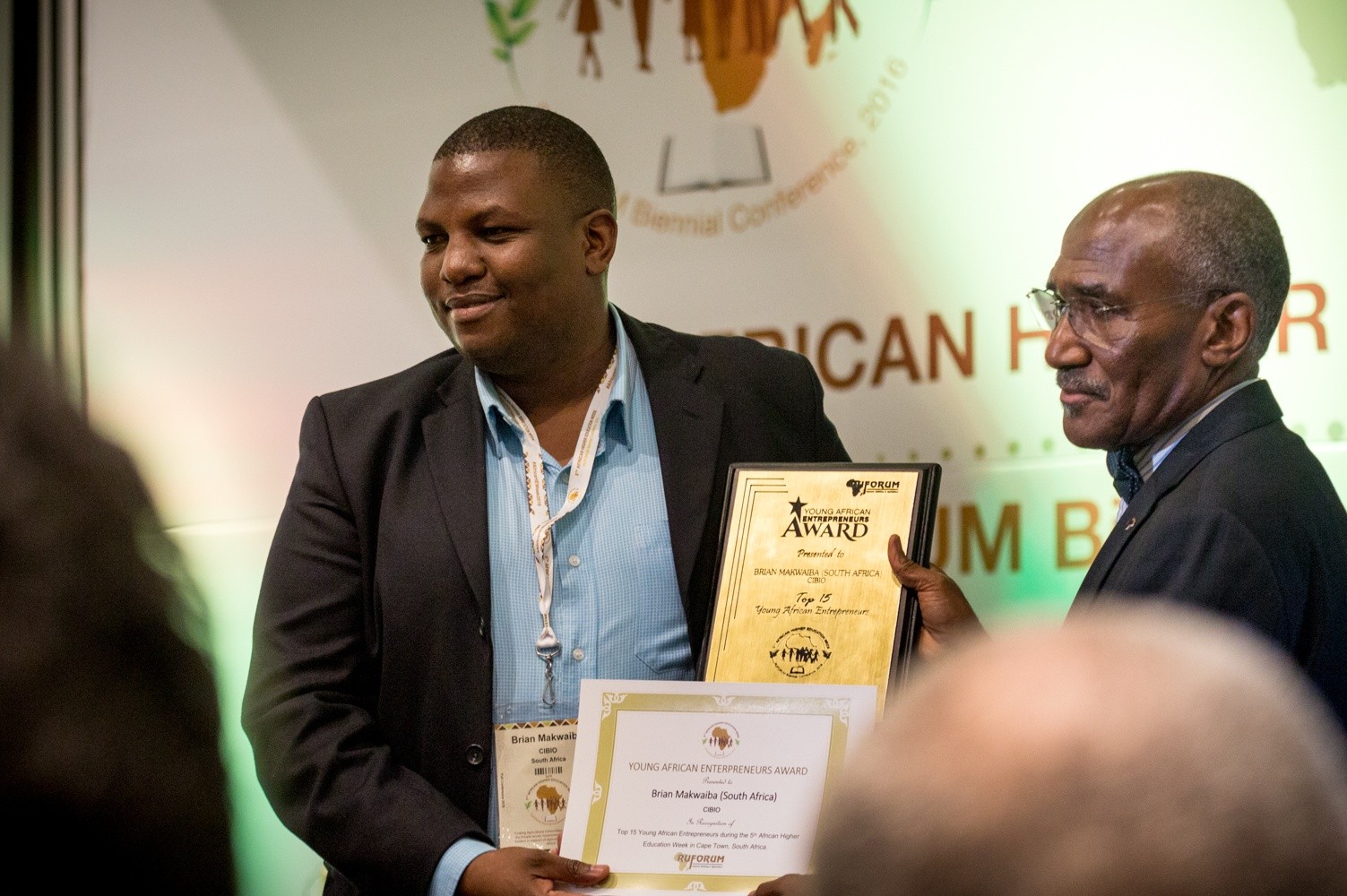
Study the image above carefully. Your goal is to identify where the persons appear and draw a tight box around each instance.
[0,334,242,896]
[804,592,1347,896]
[750,173,1347,896]
[227,107,852,896]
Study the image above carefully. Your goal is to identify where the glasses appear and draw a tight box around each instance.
[1029,287,1224,344]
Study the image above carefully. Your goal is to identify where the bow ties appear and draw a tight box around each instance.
[1107,448,1142,506]
